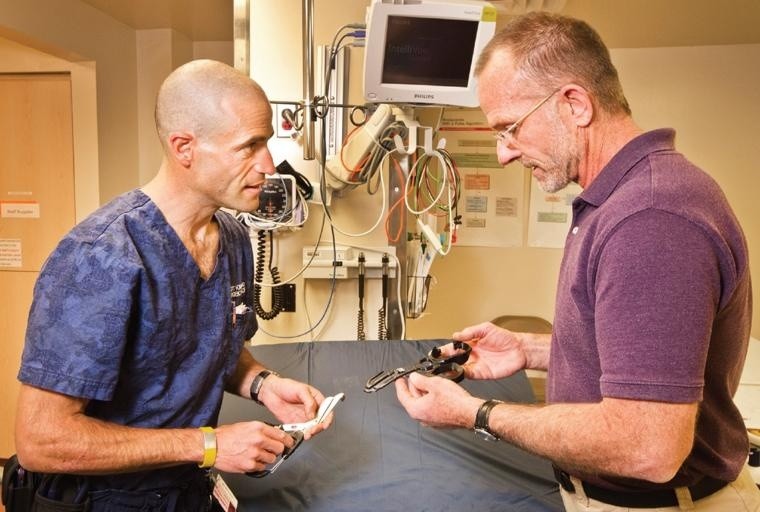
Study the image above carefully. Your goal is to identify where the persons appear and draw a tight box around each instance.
[393,11,760,512]
[14,58,335,512]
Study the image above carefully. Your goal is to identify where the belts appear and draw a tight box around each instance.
[552,464,728,508]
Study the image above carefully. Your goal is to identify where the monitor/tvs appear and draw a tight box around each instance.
[363,0,497,108]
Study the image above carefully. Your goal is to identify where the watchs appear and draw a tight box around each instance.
[473,399,504,443]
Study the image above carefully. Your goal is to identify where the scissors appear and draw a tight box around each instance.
[247,392,345,478]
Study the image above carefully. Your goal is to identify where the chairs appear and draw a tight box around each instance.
[490,316,553,406]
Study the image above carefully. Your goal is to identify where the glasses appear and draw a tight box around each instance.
[493,87,561,150]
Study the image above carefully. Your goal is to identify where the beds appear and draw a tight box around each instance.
[214,339,565,512]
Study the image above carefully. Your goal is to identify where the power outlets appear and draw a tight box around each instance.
[276,102,298,137]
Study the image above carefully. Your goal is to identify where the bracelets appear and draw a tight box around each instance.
[249,368,280,406]
[197,426,217,470]
